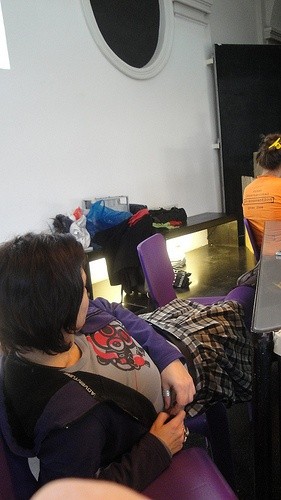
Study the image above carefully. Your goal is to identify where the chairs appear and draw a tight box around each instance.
[136,233,228,307]
[244,219,261,266]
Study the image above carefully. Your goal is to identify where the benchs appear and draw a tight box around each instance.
[84,211,242,301]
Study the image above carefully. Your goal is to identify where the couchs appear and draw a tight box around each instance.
[0,439,239,500]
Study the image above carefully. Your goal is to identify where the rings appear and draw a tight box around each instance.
[183,427,190,437]
[161,390,171,397]
[183,437,189,441]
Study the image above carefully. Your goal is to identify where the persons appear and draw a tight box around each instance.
[0,230,281,493]
[240,134,281,257]
